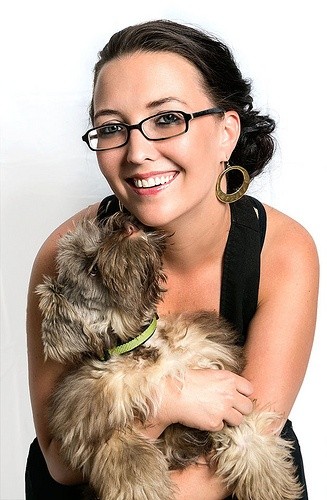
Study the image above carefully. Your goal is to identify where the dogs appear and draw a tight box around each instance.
[32,205,306,499]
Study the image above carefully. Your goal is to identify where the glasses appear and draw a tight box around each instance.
[83,106,222,152]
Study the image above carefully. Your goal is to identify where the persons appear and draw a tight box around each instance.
[19,20,322,499]
